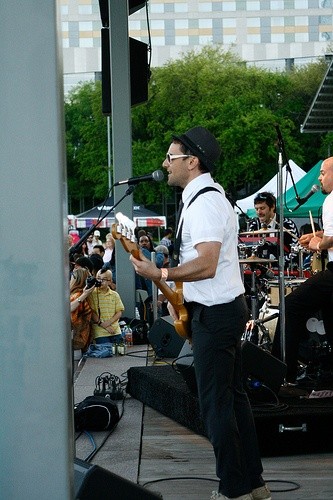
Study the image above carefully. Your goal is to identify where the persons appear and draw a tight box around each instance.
[67,228,116,291]
[85,262,125,345]
[130,126,275,500]
[69,267,115,361]
[246,192,301,270]
[298,157,333,270]
[132,226,174,327]
[295,221,322,272]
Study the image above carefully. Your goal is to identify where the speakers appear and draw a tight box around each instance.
[75,456,162,500]
[149,316,186,358]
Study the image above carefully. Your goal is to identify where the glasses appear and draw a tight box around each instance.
[164,153,192,163]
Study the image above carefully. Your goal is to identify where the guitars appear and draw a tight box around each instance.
[110,211,192,346]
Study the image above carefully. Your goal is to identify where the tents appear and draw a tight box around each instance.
[234,158,324,236]
[247,160,332,219]
[69,193,167,246]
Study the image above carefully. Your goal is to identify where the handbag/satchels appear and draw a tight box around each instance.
[74,396,120,432]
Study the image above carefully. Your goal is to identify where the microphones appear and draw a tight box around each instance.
[293,184,319,212]
[113,170,164,186]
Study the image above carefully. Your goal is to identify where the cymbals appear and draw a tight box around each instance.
[238,254,278,263]
[237,228,293,235]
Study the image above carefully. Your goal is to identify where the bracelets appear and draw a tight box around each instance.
[160,268,168,282]
[77,298,82,304]
[317,242,322,252]
[98,320,104,326]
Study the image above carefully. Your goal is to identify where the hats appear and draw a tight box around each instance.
[172,126,220,174]
[70,257,93,271]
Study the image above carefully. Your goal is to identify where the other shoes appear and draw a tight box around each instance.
[208,485,272,500]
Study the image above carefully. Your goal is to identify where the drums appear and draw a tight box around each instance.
[268,277,308,307]
[255,308,280,354]
